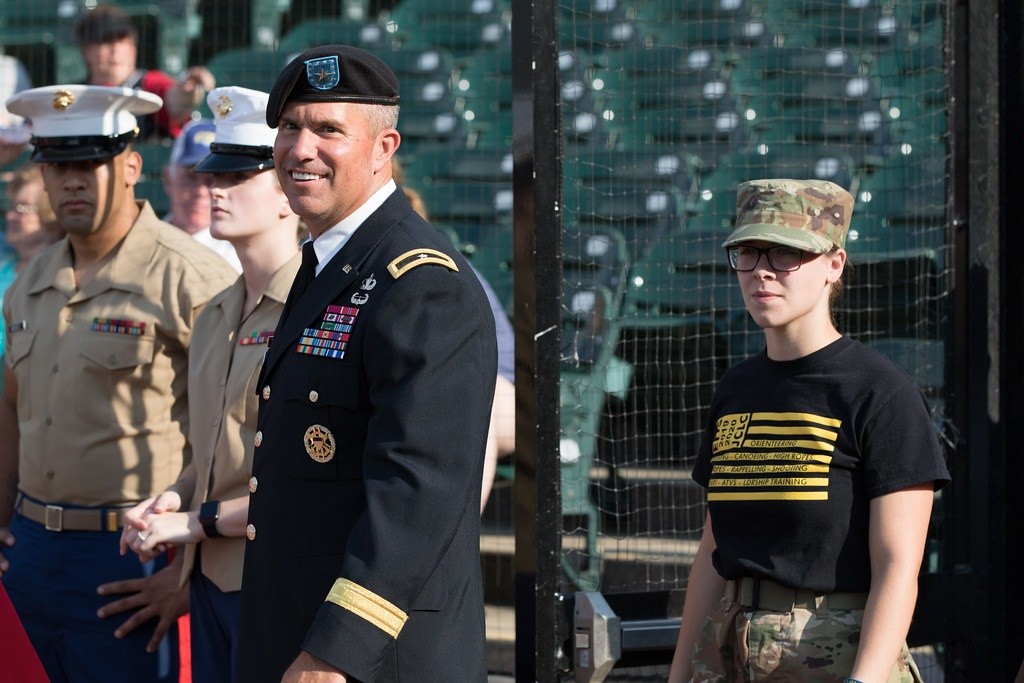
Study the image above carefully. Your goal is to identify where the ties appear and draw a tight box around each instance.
[272,241,319,350]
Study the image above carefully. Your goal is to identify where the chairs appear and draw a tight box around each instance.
[0,0,951,577]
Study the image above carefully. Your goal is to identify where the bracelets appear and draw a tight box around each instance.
[843,678,863,683]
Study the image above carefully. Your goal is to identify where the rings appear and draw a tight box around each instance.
[137,531,146,541]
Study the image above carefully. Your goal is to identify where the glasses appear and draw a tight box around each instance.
[726,243,835,272]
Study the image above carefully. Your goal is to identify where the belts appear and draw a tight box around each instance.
[723,578,870,612]
[14,490,135,532]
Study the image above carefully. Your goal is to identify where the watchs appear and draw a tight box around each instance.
[199,500,226,539]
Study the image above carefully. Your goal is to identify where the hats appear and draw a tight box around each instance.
[76,7,135,40]
[190,87,278,174]
[266,46,401,129]
[722,179,855,255]
[6,85,163,163]
[171,117,216,167]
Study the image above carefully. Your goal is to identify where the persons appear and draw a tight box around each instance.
[0,5,516,682]
[667,178,951,683]
